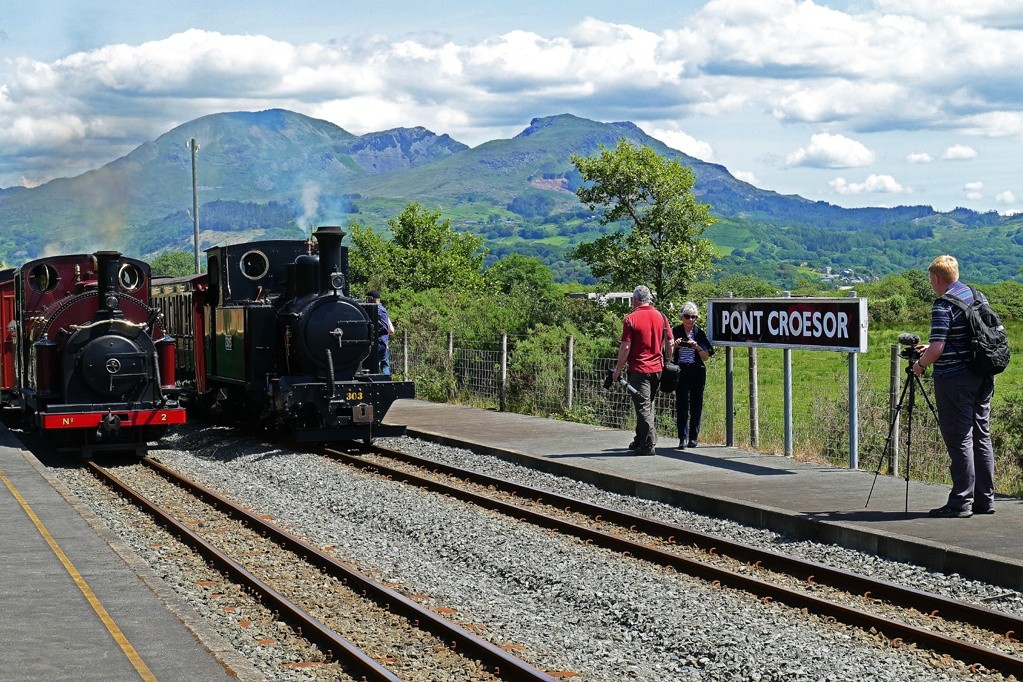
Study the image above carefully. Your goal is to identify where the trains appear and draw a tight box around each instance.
[0,248,187,461]
[150,223,416,450]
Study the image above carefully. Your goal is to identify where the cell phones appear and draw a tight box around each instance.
[678,341,692,348]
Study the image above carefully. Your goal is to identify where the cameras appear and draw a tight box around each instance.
[603,369,614,390]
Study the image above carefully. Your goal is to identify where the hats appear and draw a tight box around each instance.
[365,290,381,298]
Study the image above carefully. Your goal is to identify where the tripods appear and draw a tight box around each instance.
[865,361,938,516]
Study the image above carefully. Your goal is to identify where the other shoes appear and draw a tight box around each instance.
[930,505,973,517]
[629,442,641,450]
[636,445,655,456]
[977,508,996,514]
[687,440,697,447]
[679,441,686,449]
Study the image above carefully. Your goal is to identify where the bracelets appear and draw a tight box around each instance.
[698,347,703,352]
[918,358,925,367]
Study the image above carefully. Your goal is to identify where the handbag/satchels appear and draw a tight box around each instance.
[660,364,680,392]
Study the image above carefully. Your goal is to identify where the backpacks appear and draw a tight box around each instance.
[934,285,1010,373]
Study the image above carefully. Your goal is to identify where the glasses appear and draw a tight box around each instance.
[682,314,697,320]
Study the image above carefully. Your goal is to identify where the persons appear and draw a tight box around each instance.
[913,254,996,519]
[671,302,715,451]
[366,290,395,375]
[612,285,675,456]
